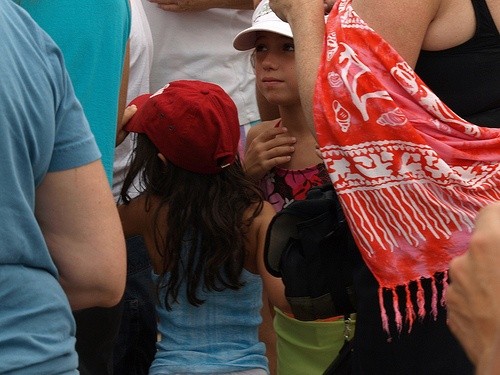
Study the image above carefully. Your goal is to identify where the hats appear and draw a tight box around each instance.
[123,80,242,173]
[233,0,328,51]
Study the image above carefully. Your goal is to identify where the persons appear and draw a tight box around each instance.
[13,0,500,375]
[0,0,127,375]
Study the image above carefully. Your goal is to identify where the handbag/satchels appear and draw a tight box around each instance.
[264,183,379,321]
